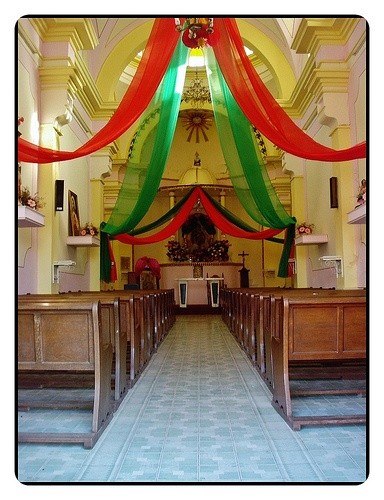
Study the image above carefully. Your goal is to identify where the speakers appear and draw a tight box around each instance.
[330,176,338,208]
[55,179,64,211]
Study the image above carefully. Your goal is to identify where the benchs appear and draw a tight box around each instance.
[18,284,369,451]
